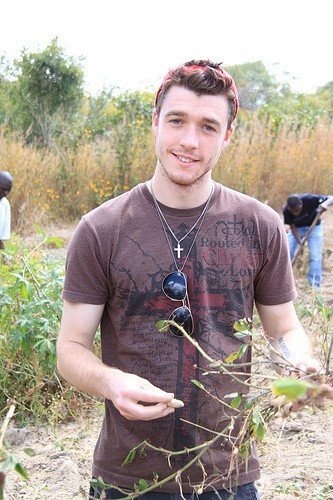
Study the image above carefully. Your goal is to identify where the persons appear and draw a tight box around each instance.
[0,171,13,250]
[55,59,333,498]
[282,194,333,287]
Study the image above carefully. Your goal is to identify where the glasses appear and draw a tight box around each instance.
[161,270,195,338]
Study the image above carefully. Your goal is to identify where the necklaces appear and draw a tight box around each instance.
[153,188,211,272]
